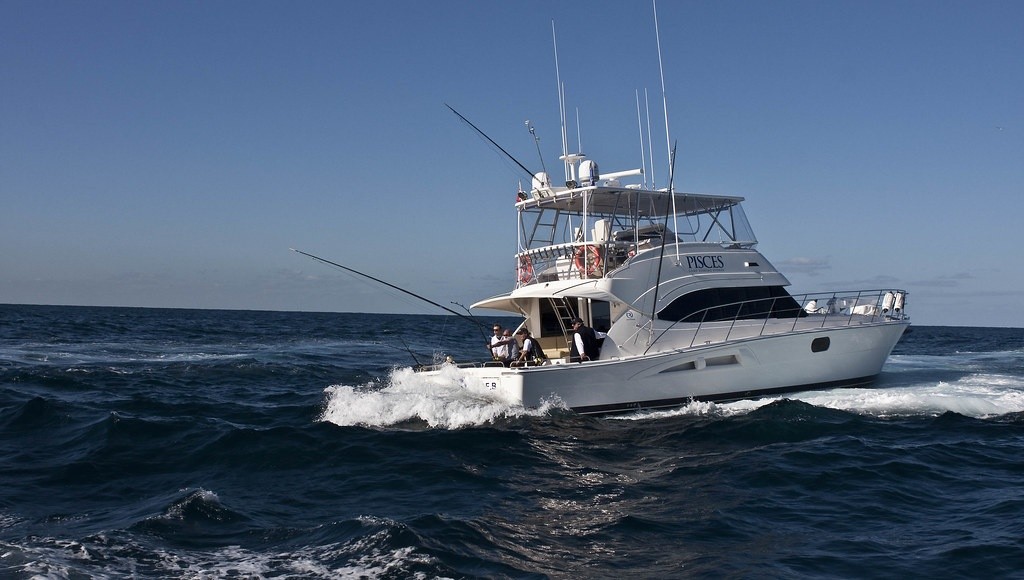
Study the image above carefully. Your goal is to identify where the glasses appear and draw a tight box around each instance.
[493,329,498,331]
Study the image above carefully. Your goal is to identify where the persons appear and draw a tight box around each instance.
[486,323,519,366]
[826,297,836,313]
[627,243,636,258]
[509,328,546,366]
[804,299,819,314]
[570,317,607,362]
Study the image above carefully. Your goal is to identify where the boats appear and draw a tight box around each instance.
[412,0,912,419]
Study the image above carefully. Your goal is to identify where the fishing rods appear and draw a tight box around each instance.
[442,103,545,186]
[645,137,677,335]
[290,247,493,330]
[382,301,538,363]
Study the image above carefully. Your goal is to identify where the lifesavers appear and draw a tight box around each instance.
[575,245,600,274]
[517,256,533,283]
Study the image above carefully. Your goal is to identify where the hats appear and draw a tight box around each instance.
[516,328,528,334]
[571,317,584,323]
